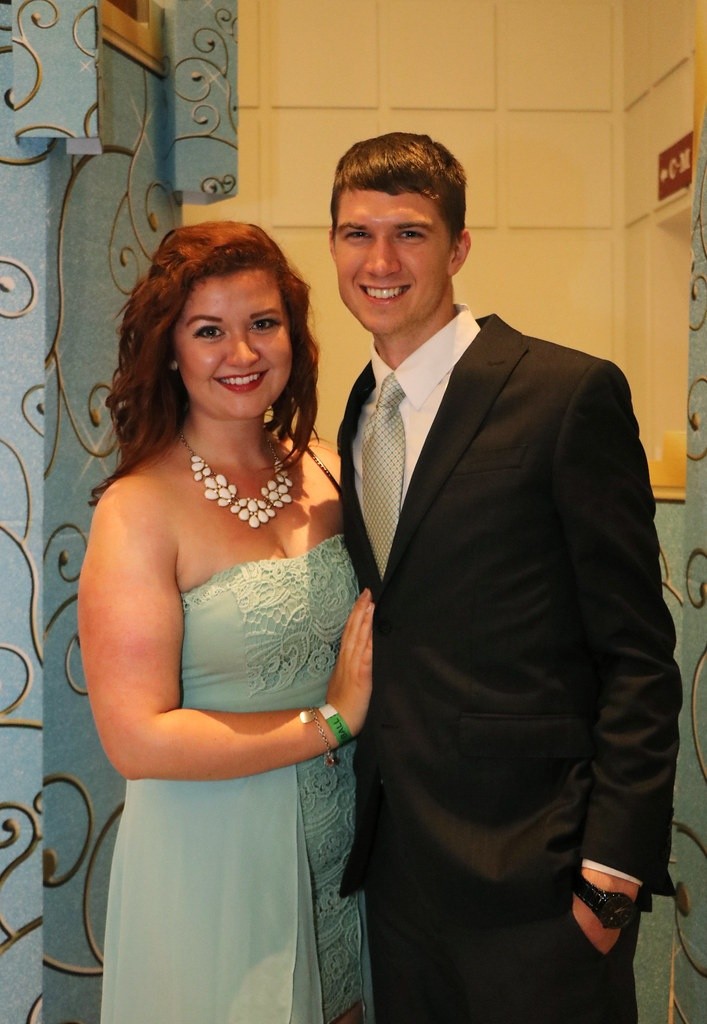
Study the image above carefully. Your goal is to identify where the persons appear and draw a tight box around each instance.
[329,132,682,1024]
[78,221,375,1024]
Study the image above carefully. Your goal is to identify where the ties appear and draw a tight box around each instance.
[363,372,406,579]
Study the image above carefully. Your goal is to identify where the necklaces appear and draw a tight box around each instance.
[180,428,293,528]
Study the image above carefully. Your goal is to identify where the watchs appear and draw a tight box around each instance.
[573,873,637,930]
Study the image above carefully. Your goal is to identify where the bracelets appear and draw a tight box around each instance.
[300,706,335,768]
[318,704,354,746]
